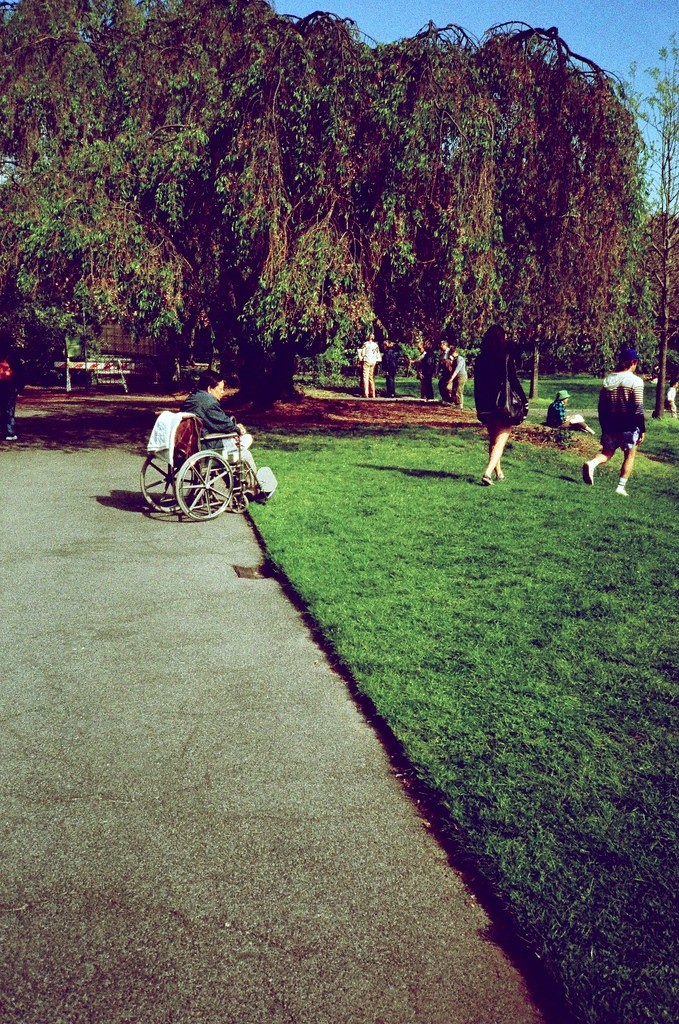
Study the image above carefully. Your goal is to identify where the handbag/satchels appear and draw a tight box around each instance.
[493,346,524,425]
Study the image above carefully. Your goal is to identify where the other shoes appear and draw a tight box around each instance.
[583,462,593,485]
[495,473,505,480]
[5,436,17,440]
[615,488,629,496]
[455,405,463,409]
[481,474,494,485]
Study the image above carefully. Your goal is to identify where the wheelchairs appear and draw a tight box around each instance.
[138,409,275,523]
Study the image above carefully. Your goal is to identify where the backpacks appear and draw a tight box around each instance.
[0,354,14,381]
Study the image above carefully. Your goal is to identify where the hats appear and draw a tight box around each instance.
[618,349,640,362]
[555,390,570,401]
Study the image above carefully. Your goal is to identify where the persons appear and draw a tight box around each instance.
[583,349,647,496]
[181,370,258,475]
[0,342,24,440]
[360,332,399,398]
[664,380,679,418]
[474,323,529,485]
[546,390,596,435]
[410,340,468,409]
